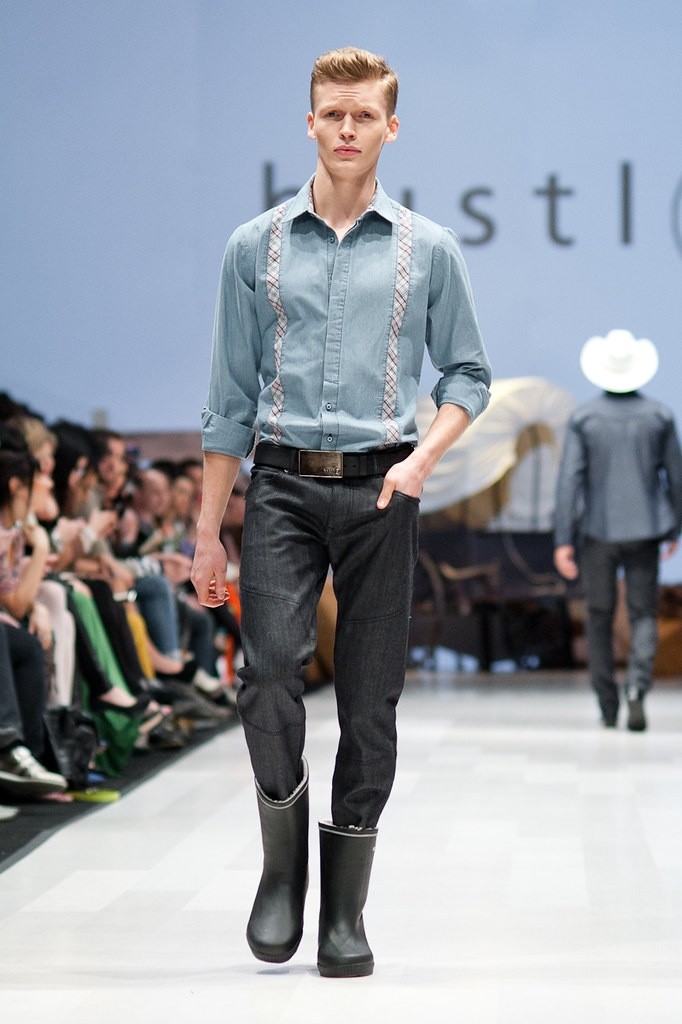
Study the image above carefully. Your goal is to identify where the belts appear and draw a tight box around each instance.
[254,440,414,478]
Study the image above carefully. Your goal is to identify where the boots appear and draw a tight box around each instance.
[246,755,309,962]
[316,819,379,978]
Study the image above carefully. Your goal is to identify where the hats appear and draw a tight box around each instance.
[579,328,660,394]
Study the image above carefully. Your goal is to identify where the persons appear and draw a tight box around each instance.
[186,44,496,973]
[552,326,682,739]
[1,380,584,878]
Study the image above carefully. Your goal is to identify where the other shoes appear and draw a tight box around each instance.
[626,686,646,731]
[600,680,619,725]
[0,647,245,821]
